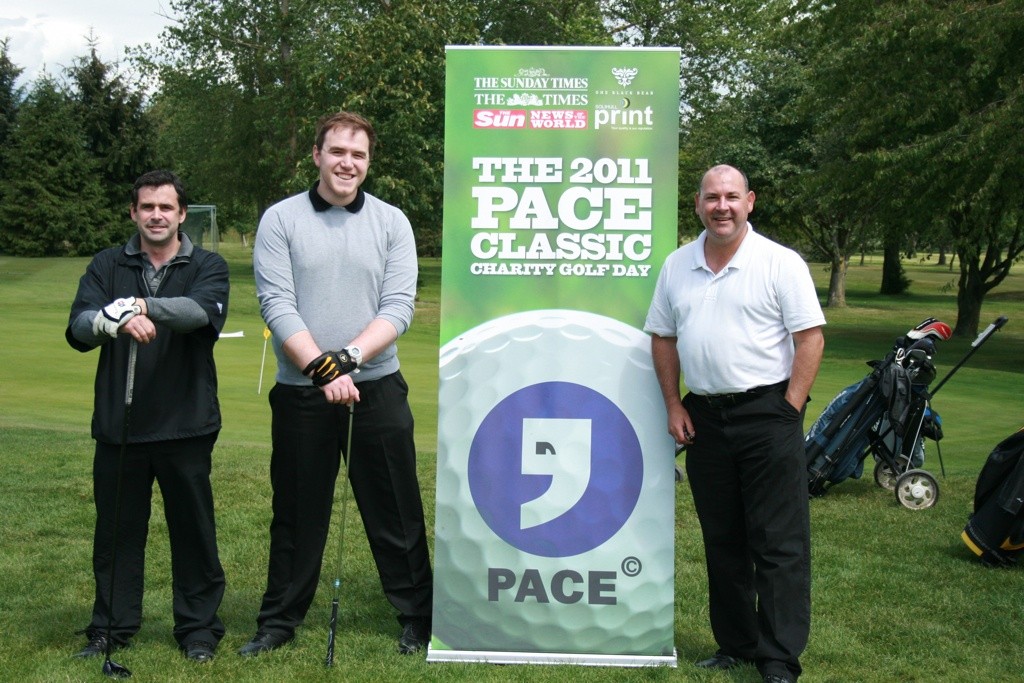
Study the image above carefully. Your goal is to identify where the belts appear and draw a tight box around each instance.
[697,386,776,409]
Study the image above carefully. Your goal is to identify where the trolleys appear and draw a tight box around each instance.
[802,315,1010,512]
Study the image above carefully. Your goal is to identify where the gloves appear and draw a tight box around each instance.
[92,296,142,339]
[302,349,357,386]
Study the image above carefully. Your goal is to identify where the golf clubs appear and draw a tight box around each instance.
[101,334,139,678]
[324,396,355,666]
[901,348,936,374]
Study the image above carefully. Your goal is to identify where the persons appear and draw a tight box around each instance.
[644,164,827,683]
[237,113,434,658]
[65,169,230,664]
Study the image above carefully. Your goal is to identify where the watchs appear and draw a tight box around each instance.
[344,345,363,367]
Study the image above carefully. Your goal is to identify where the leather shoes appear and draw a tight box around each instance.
[397,621,428,655]
[184,641,215,663]
[71,631,126,658]
[763,674,793,683]
[694,652,752,670]
[237,633,294,657]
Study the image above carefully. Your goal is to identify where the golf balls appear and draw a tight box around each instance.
[434,307,675,657]
